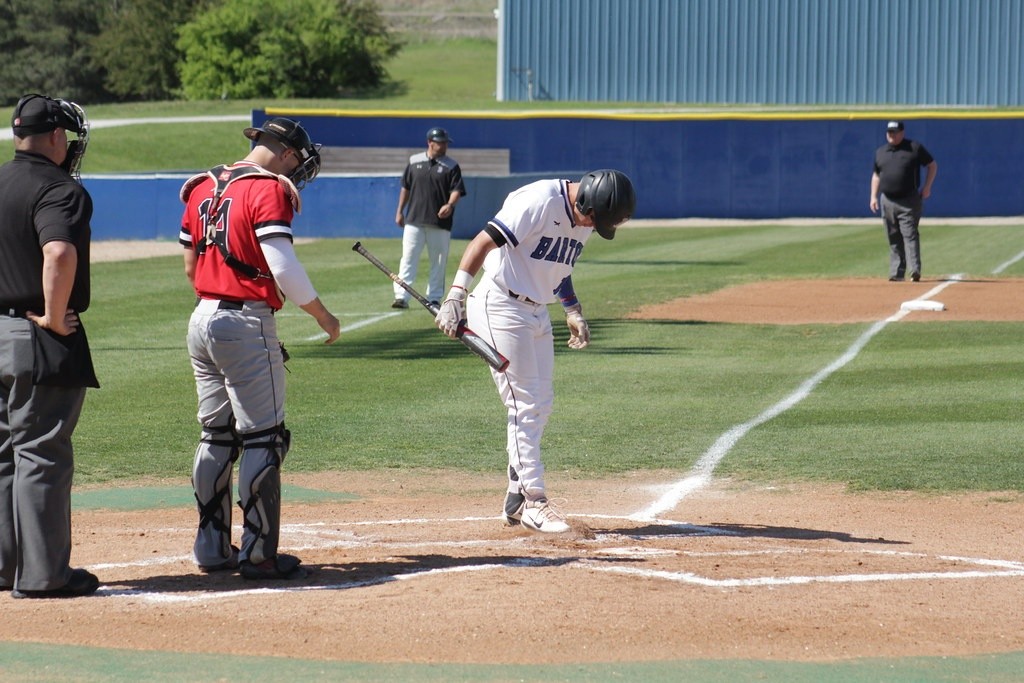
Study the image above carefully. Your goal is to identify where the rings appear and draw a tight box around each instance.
[445,215,448,217]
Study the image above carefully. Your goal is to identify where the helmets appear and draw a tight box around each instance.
[426,126,453,143]
[11,94,84,134]
[574,169,636,240]
[243,116,320,180]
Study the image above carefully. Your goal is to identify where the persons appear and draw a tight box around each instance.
[392,129,467,309]
[870,119,936,283]
[435,171,636,533]
[180,118,341,580]
[0,92,101,599]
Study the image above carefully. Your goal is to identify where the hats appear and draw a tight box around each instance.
[886,120,904,132]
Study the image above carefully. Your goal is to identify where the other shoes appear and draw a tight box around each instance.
[888,277,905,281]
[392,299,409,308]
[910,271,921,281]
[430,301,440,310]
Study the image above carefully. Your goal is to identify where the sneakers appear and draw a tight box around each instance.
[504,502,572,533]
[0,568,99,599]
[240,554,308,580]
[199,545,242,573]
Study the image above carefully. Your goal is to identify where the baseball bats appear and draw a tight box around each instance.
[351,239,510,373]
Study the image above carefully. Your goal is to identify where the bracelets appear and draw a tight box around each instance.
[447,203,456,210]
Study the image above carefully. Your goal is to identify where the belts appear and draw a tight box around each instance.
[509,289,535,304]
